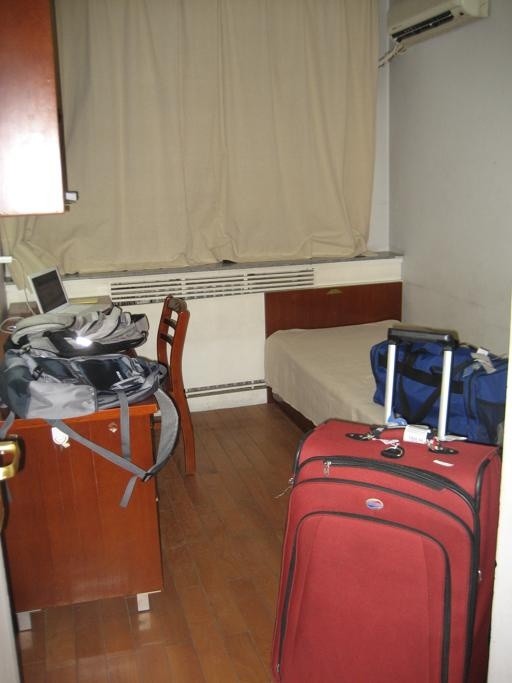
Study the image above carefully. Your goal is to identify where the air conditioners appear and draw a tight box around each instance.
[387,1,489,49]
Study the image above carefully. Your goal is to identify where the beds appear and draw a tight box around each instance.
[265,282,417,424]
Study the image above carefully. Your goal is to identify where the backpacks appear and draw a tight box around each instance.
[1,346,179,510]
[12,300,150,357]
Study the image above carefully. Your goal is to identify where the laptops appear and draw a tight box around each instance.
[27,265,113,315]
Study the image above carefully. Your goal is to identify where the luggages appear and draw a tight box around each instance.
[271,328,502,683]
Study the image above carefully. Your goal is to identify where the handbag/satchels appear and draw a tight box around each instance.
[372,325,509,439]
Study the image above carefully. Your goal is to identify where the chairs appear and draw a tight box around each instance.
[156,295,196,477]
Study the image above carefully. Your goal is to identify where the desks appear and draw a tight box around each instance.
[0,295,164,632]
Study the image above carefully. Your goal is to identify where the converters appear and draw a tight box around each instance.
[0,256,13,263]
